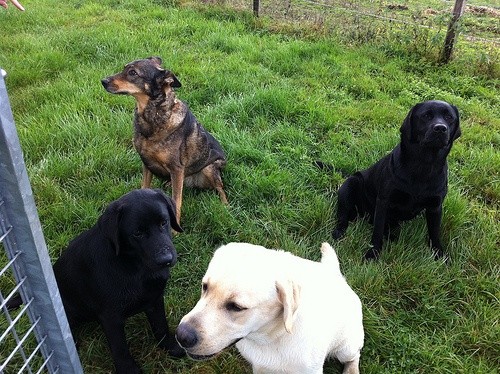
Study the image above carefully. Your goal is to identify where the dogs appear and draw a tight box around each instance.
[175,240,365,374]
[0,188,186,374]
[331,100,461,267]
[101,56,229,240]
